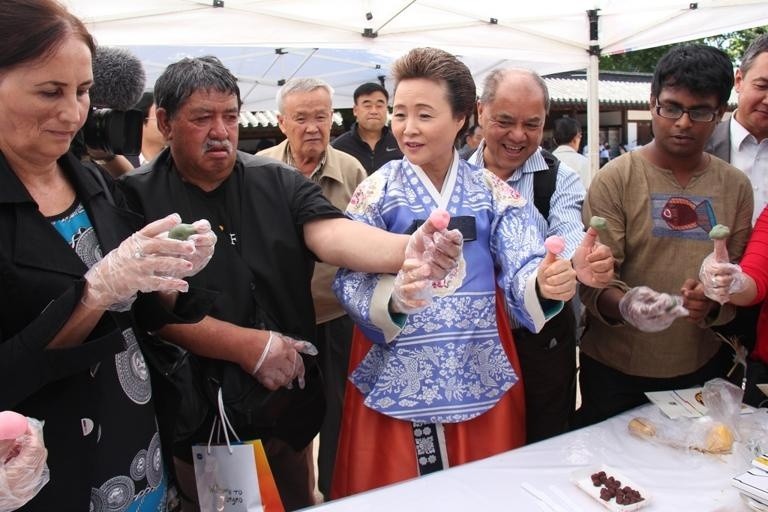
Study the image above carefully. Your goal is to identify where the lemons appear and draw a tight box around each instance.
[707,426,733,452]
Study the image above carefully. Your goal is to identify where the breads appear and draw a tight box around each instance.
[628,417,656,438]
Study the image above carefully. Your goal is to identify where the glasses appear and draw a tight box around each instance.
[654,97,724,125]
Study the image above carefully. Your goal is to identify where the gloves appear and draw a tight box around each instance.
[252,328,320,391]
[404,207,465,285]
[391,257,433,317]
[79,211,219,311]
[697,223,750,306]
[0,408,52,512]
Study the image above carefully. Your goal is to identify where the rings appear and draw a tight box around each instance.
[134,239,144,259]
[711,277,717,288]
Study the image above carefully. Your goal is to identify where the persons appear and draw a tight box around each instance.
[699,201,768,401]
[618,144,626,154]
[125,92,169,169]
[116,55,462,512]
[578,44,755,420]
[703,33,768,230]
[459,68,614,446]
[459,124,483,162]
[327,47,578,505]
[599,142,611,169]
[249,76,370,503]
[550,117,591,192]
[1,0,218,511]
[329,82,406,178]
[79,107,134,179]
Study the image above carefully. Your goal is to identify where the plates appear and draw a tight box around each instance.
[571,466,651,511]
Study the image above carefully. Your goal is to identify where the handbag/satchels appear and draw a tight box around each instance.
[190,384,289,512]
[618,285,691,334]
[82,158,219,441]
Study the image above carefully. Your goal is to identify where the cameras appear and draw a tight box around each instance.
[70,105,143,159]
